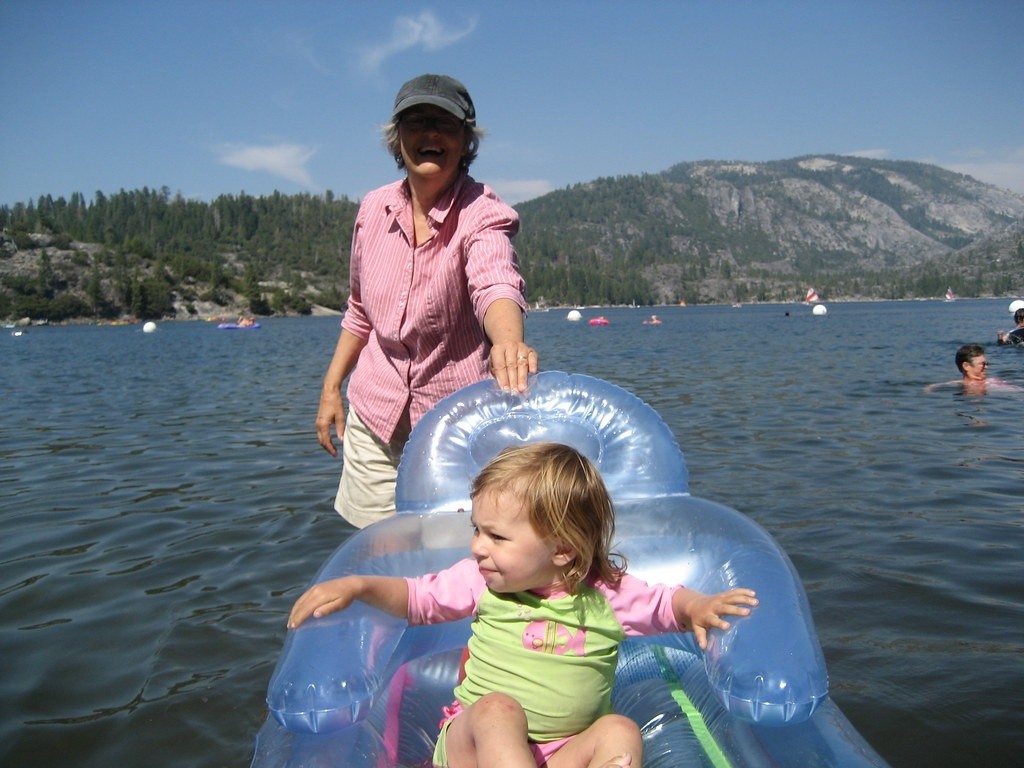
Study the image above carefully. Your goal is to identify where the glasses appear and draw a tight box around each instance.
[403,113,465,136]
[981,361,989,367]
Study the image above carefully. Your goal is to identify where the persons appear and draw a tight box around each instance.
[236,312,256,326]
[285,443,759,768]
[956,345,988,381]
[316,74,538,553]
[997,300,1024,347]
[651,315,658,323]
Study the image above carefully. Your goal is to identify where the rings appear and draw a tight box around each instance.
[518,357,527,359]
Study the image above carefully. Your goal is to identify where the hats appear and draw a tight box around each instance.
[393,74,476,125]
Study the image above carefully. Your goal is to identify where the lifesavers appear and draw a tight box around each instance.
[590,319,609,325]
